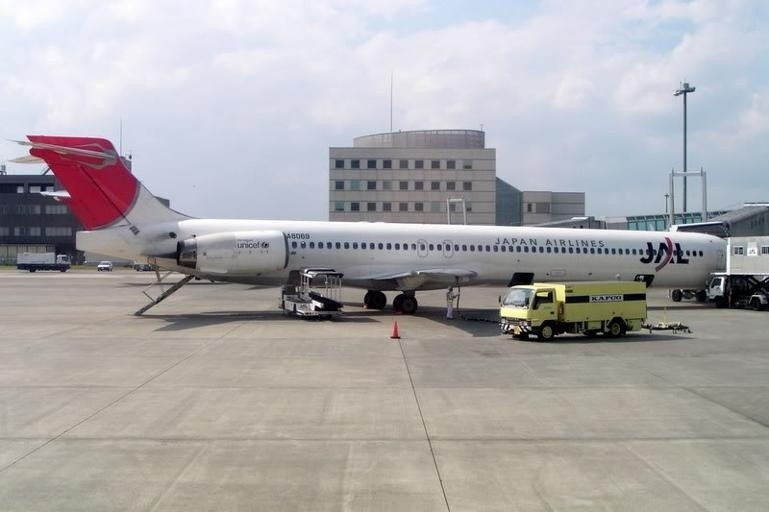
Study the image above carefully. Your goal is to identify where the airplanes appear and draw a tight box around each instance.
[1,125,732,316]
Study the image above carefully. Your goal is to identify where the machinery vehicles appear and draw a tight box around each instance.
[704,270,769,311]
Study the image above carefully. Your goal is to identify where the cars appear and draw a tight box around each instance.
[96,259,114,273]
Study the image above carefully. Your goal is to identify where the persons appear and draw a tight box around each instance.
[446,285,461,322]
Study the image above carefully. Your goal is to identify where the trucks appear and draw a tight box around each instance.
[132,260,160,271]
[492,277,651,342]
[14,251,73,273]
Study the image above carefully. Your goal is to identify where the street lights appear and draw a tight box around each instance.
[672,80,697,211]
[664,190,670,214]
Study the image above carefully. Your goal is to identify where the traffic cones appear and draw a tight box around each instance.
[390,320,403,340]
